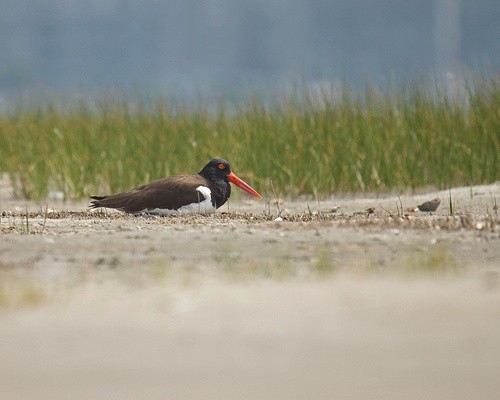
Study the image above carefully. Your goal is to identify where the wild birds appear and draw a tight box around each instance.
[416,197,441,216]
[88,157,263,218]
